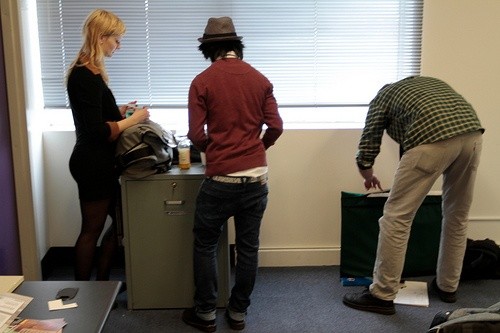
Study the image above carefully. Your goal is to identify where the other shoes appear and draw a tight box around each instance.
[224,309,246,330]
[181,312,217,333]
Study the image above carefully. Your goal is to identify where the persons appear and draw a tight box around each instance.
[65,9,150,292]
[343,75,484,314]
[179,17,283,333]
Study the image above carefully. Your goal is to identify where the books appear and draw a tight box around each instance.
[0,276,33,331]
[393,280,430,308]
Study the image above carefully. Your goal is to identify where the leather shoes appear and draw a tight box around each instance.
[342,287,396,315]
[425,277,457,304]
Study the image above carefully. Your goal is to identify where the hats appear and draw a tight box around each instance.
[197,16,243,43]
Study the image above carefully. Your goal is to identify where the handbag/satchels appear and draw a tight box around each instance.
[113,117,174,180]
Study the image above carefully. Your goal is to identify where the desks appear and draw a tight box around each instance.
[10,281,122,333]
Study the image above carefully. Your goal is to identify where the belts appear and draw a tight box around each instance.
[212,175,267,184]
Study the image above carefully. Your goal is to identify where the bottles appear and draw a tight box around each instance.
[177,136,191,169]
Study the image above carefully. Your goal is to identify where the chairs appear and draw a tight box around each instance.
[426,302,500,333]
